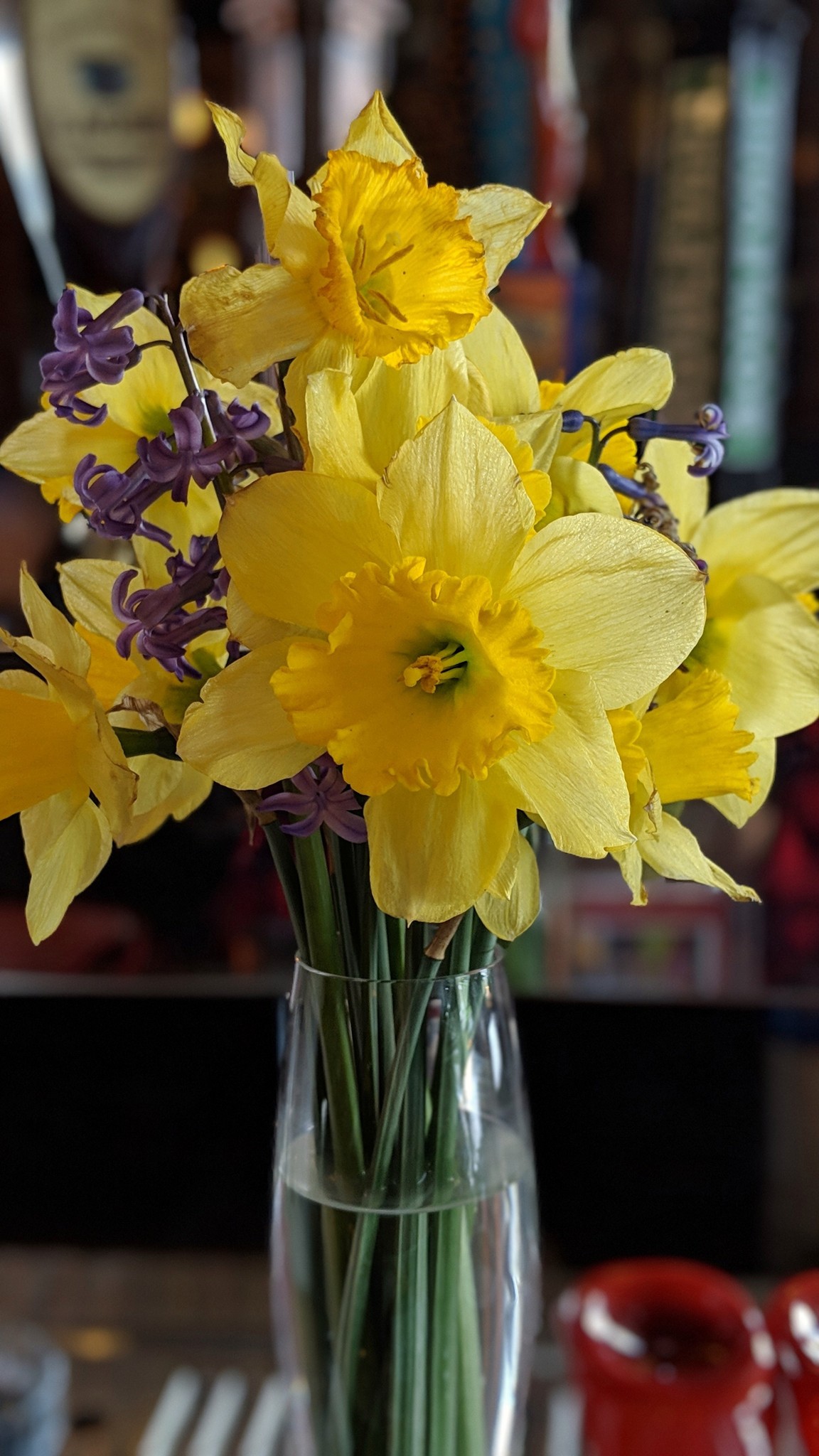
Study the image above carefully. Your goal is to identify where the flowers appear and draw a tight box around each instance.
[0,89,819,950]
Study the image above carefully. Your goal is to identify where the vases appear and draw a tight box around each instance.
[268,952,545,1456]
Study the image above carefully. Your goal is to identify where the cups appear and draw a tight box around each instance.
[766,1270,818,1456]
[556,1257,778,1456]
